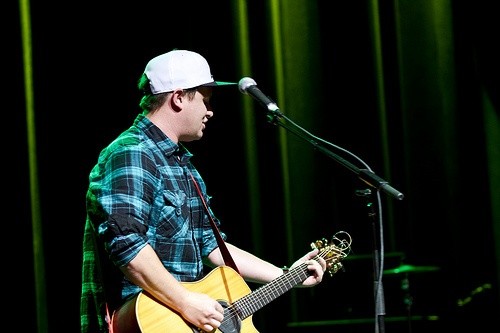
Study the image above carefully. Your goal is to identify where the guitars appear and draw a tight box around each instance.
[112,231,352,333]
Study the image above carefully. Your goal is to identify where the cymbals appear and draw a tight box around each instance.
[383,264,438,274]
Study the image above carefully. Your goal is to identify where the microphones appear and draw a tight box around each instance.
[238,76,283,118]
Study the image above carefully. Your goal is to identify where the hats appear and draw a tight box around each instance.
[138,49,239,95]
